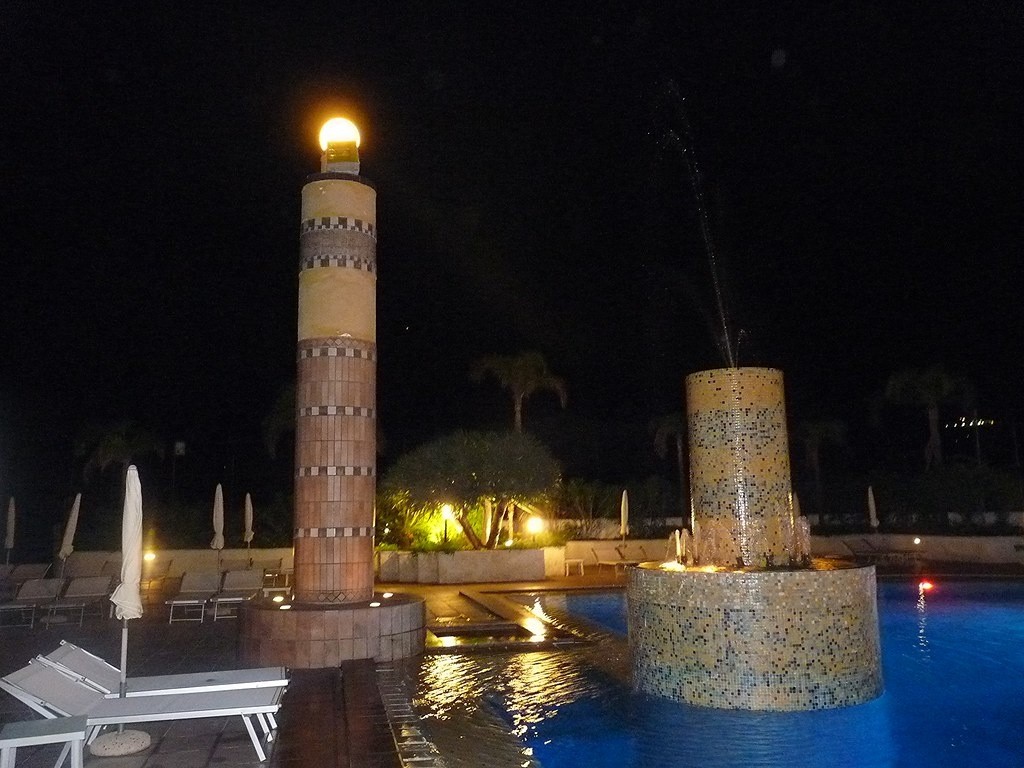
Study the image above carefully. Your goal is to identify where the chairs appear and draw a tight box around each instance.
[841,538,928,567]
[590,545,665,573]
[0,557,297,629]
[0,640,288,762]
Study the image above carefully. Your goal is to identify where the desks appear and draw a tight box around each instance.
[263,586,291,597]
[565,559,584,577]
[0,714,88,768]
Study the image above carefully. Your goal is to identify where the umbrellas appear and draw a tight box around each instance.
[109,465,143,735]
[868,486,879,527]
[4,496,15,571]
[620,490,629,558]
[55,492,82,600]
[245,493,254,569]
[211,483,225,594]
